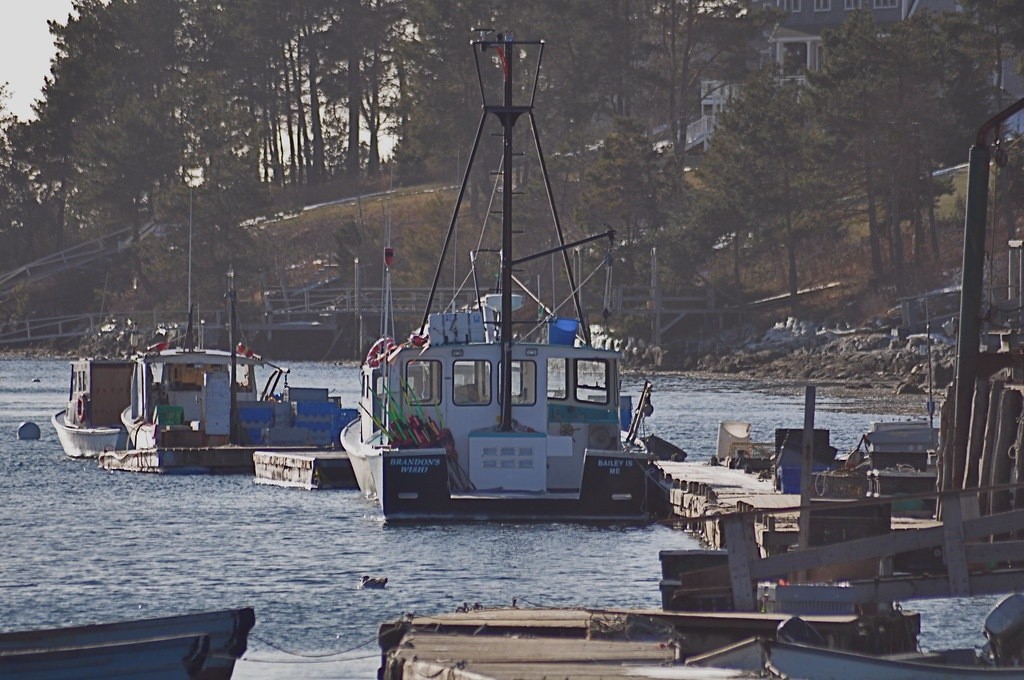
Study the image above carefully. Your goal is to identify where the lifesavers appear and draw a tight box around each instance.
[366,336,395,368]
[76,394,87,424]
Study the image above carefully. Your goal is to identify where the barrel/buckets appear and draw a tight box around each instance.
[868,450,937,495]
[17,422,41,441]
[546,316,579,346]
[778,466,869,499]
[717,421,751,459]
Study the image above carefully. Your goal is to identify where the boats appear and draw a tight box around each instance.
[339,28,661,526]
[51,183,362,474]
[752,591,1024,680]
[860,287,940,470]
